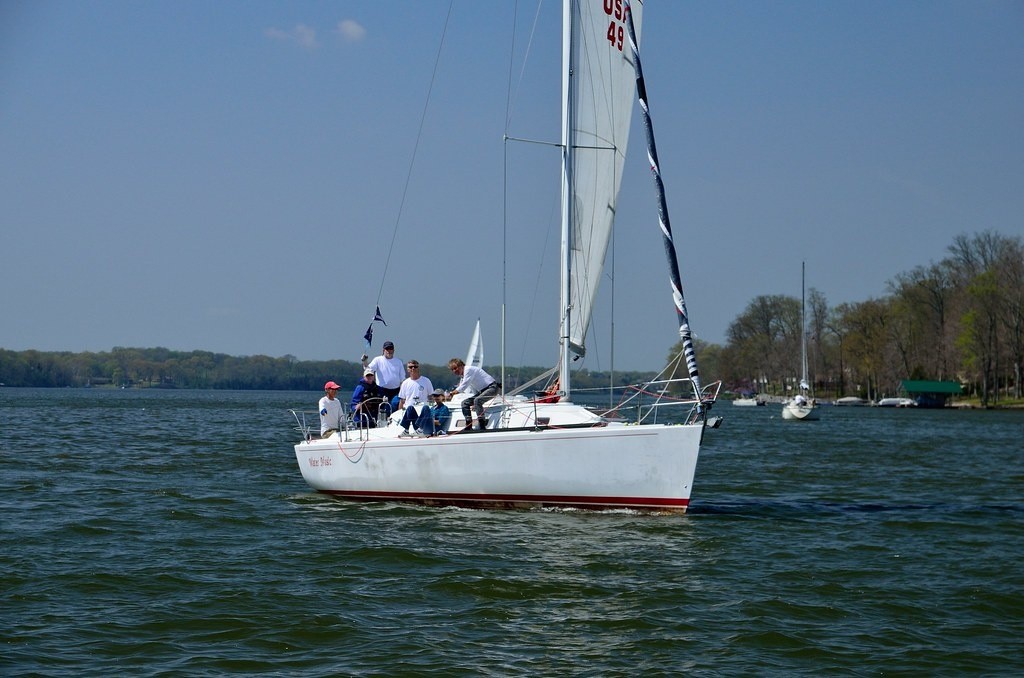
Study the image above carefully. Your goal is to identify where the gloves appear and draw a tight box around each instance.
[321,409,327,416]
[361,354,368,362]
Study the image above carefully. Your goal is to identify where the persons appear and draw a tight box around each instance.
[350,369,380,430]
[397,360,449,438]
[361,341,406,424]
[445,358,499,434]
[319,381,349,439]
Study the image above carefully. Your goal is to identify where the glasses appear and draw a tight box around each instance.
[433,395,440,397]
[409,366,418,369]
[452,367,457,371]
[386,347,393,350]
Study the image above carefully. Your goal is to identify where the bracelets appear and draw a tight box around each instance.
[449,391,453,396]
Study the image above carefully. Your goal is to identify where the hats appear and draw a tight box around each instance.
[364,369,374,376]
[429,388,445,397]
[325,381,341,389]
[383,341,394,348]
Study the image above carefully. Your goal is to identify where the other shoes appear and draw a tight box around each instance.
[461,421,472,431]
[474,419,486,430]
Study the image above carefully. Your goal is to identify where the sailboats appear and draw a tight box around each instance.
[286,0,724,518]
[781,260,822,423]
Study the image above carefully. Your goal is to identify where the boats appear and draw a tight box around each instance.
[732,397,767,407]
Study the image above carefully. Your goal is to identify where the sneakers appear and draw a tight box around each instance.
[412,428,424,436]
[398,429,409,437]
